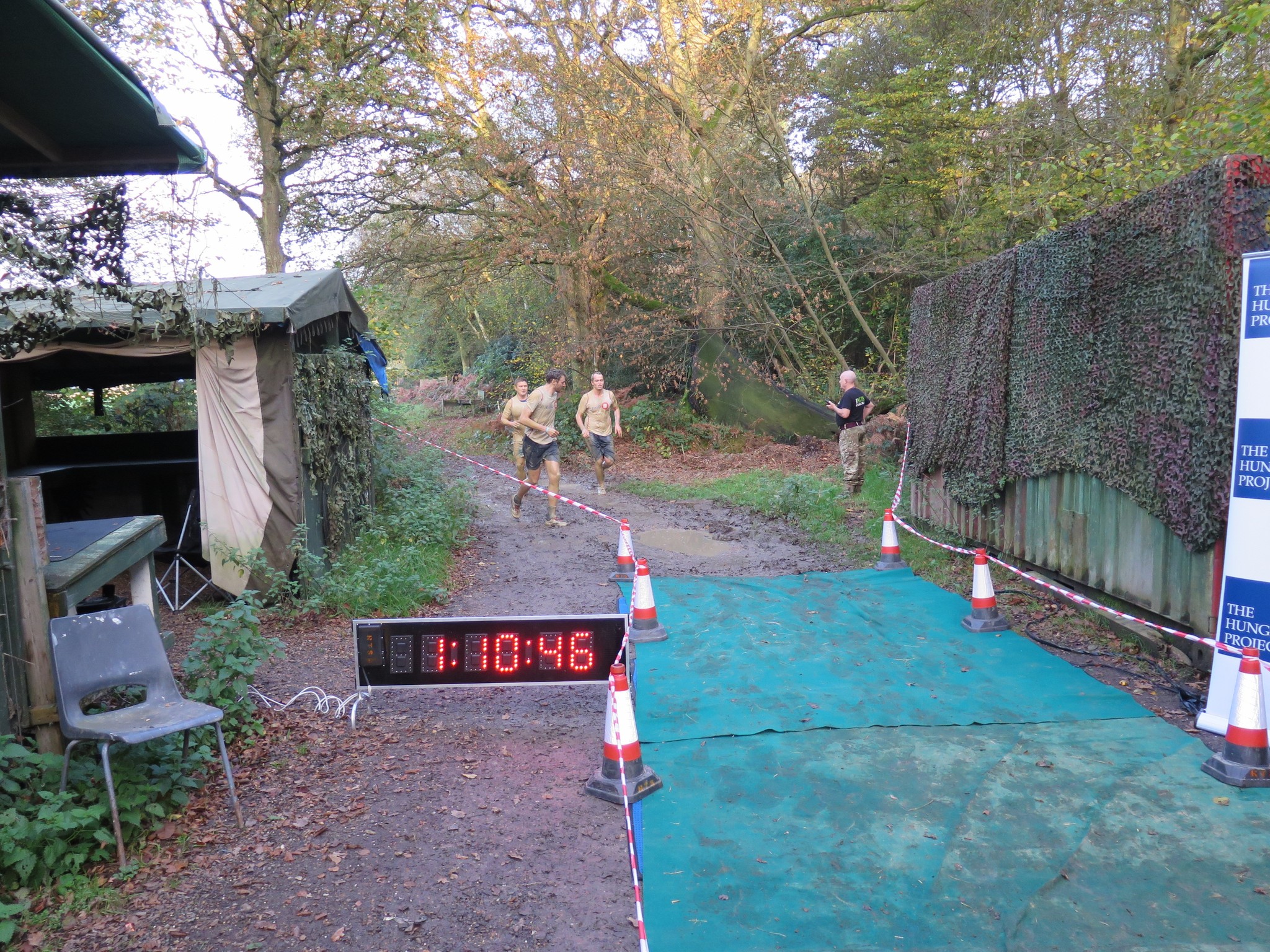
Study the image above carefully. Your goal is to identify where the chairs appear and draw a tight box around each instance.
[50,604,247,881]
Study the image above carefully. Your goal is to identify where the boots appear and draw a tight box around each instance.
[854,485,862,494]
[836,484,855,496]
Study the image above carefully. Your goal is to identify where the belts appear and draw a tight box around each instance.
[840,421,863,431]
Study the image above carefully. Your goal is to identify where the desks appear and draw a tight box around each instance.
[41,514,175,655]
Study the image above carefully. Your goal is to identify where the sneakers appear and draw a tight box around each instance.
[511,494,521,519]
[597,484,607,495]
[545,517,568,527]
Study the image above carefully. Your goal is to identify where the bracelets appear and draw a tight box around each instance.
[545,427,549,433]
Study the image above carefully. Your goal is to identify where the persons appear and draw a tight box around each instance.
[508,369,574,527]
[826,371,875,494]
[500,376,537,495]
[576,371,623,494]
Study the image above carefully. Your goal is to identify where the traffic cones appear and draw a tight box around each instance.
[609,518,639,581]
[1200,648,1270,788]
[584,663,661,802]
[871,508,908,572]
[961,547,1013,633]
[625,558,667,642]
